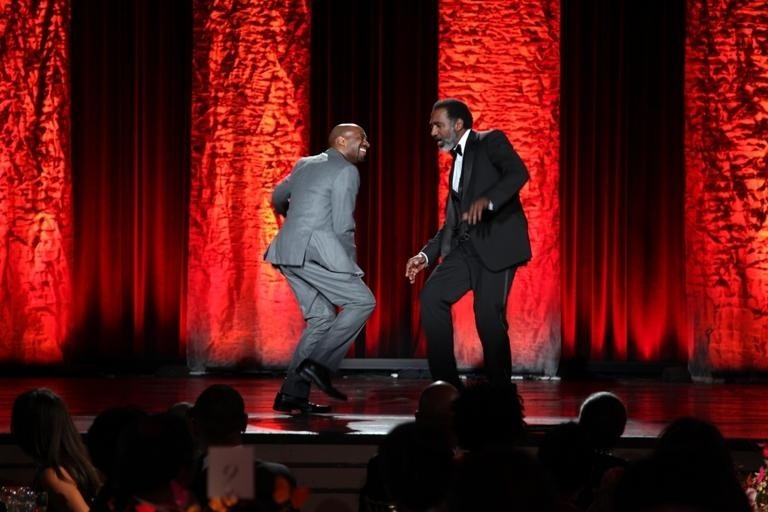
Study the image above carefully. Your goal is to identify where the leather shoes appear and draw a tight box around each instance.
[273,393,330,413]
[297,359,347,401]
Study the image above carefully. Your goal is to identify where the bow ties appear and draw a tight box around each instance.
[450,145,462,156]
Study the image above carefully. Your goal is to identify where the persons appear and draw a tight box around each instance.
[4,374,296,512]
[256,122,378,418]
[357,377,760,512]
[402,98,533,395]
